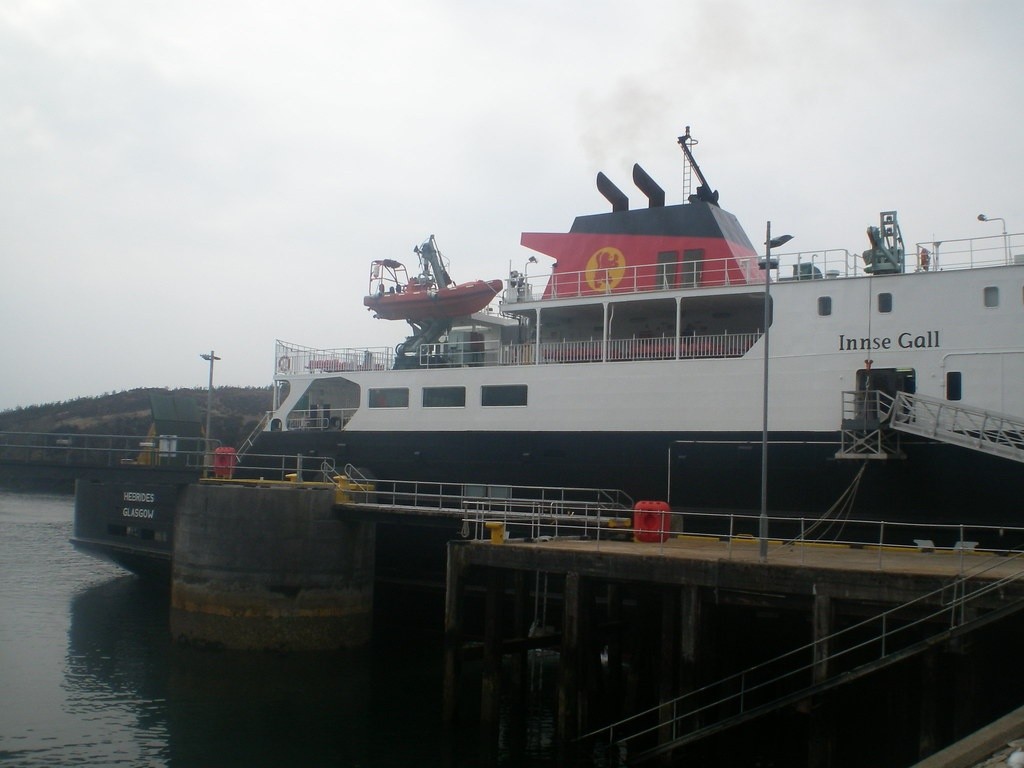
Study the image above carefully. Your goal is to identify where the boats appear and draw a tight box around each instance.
[71,128,1023,613]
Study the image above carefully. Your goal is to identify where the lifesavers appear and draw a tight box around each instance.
[920,248,932,271]
[633,497,674,542]
[212,445,237,478]
[278,355,292,372]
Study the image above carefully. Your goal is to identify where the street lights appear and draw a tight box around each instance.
[200,352,221,476]
[978,215,1008,266]
